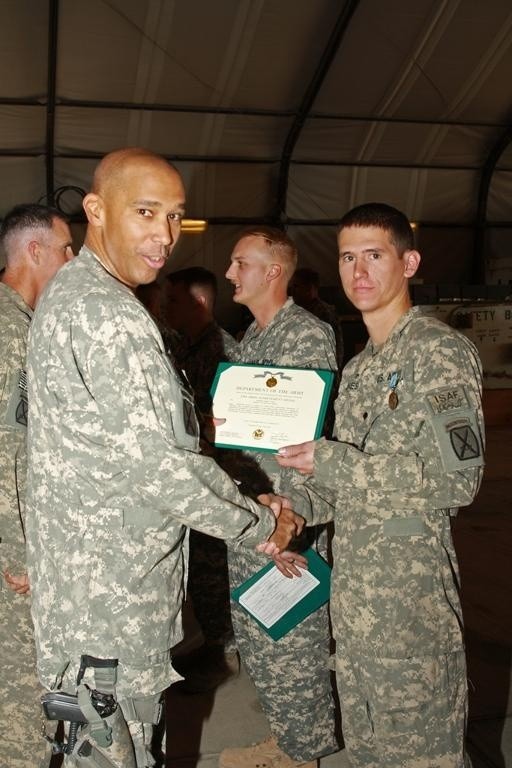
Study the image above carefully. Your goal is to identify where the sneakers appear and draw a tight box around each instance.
[175,651,239,693]
[218,736,317,768]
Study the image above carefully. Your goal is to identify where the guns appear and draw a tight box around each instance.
[40,690,116,754]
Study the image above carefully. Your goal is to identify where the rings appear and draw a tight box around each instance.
[291,559,295,564]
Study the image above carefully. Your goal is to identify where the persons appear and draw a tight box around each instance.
[24,145,304,768]
[205,228,340,768]
[256,201,489,767]
[159,266,239,696]
[0,203,77,768]
